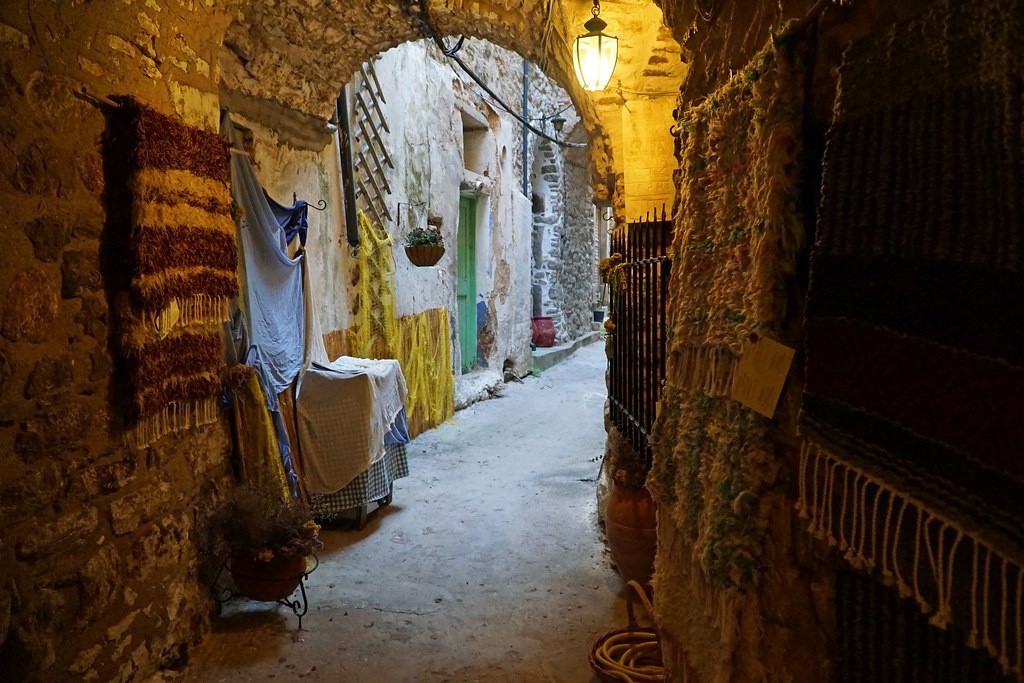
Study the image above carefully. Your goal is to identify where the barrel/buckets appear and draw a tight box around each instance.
[594,311,604,322]
[605,483,657,582]
[530,316,555,347]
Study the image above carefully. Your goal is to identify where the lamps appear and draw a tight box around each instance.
[571,0,618,91]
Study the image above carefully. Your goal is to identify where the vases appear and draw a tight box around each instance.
[229,555,307,602]
[532,316,556,347]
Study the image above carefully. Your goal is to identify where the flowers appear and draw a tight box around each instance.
[200,451,325,569]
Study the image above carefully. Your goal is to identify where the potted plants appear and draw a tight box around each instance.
[593,303,604,322]
[404,227,446,266]
